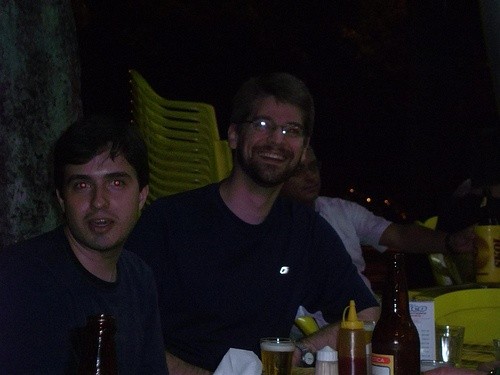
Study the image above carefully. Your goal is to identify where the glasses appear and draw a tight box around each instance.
[289,159,323,178]
[231,116,308,137]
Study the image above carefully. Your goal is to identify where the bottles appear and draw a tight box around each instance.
[337,300,366,375]
[78,313,118,375]
[472,191,500,283]
[371,249,420,375]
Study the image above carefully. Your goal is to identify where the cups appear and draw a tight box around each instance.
[259,337,296,375]
[435,325,464,368]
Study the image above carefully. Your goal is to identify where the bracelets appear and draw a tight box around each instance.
[444,234,457,254]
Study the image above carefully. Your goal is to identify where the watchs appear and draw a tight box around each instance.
[295,340,316,368]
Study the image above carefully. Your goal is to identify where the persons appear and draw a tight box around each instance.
[283,144,479,304]
[0,116,170,375]
[125,71,381,375]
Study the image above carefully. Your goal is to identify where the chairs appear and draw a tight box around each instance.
[130,72,234,205]
[429,288,500,362]
